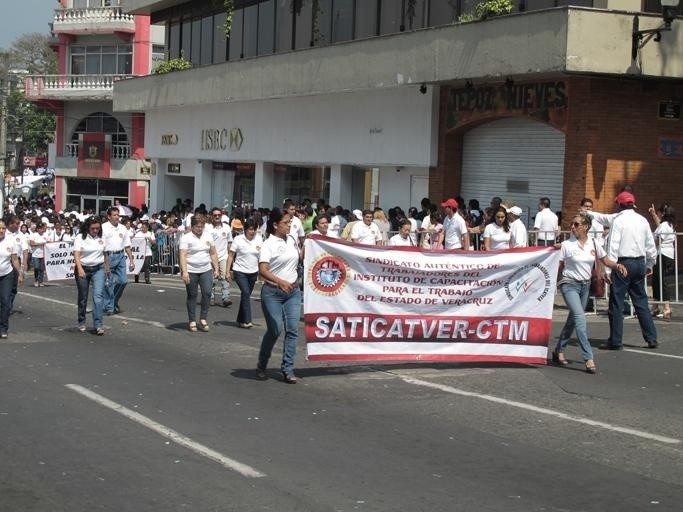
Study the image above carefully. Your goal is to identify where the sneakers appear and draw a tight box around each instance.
[34,280,45,287]
[255,367,269,381]
[0,331,8,339]
[598,338,659,350]
[80,325,105,335]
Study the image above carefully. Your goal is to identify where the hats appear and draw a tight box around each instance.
[504,205,523,217]
[353,209,363,221]
[231,220,243,229]
[614,190,636,207]
[440,197,459,210]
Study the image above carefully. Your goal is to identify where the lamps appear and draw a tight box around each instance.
[632,0,680,60]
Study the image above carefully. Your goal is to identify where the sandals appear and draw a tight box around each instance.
[189,321,198,332]
[199,319,209,331]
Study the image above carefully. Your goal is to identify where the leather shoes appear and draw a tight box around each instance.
[210,298,254,328]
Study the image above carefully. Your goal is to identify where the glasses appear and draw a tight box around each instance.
[570,222,585,227]
[279,220,293,224]
[88,216,95,222]
[213,214,221,218]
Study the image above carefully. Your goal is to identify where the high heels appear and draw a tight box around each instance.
[280,366,297,384]
[556,352,568,365]
[650,308,660,316]
[661,309,673,320]
[584,360,597,374]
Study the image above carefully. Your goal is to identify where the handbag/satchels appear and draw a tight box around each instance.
[589,267,606,299]
